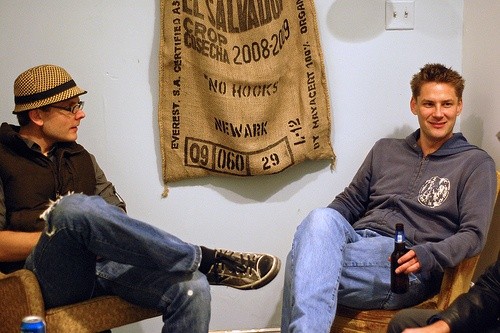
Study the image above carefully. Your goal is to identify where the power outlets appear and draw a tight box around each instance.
[385,0,415,30]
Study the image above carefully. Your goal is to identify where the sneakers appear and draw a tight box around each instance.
[204,247,281,290]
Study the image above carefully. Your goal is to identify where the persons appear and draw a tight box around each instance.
[282,63,499,332]
[1,64,282,333]
[388,253,499,332]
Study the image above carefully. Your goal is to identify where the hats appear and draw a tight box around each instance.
[11,64,87,114]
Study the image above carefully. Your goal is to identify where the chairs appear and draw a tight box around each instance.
[0,269,163,333]
[330,167,500,333]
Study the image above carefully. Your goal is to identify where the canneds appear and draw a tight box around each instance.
[18,315,47,333]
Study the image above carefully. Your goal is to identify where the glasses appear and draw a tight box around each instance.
[39,100,86,113]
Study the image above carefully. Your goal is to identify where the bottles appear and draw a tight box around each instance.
[390,224,410,295]
